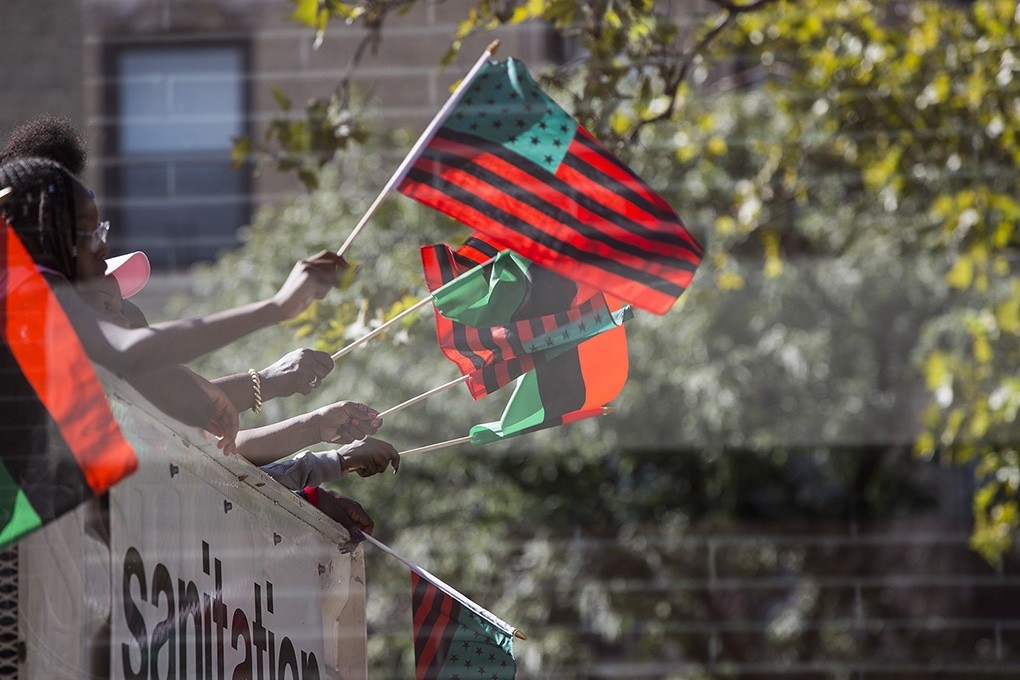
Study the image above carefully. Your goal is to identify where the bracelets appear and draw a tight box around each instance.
[248,368,262,415]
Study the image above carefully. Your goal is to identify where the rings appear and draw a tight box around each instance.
[309,375,317,388]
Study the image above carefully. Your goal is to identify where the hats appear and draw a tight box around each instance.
[104,250,151,300]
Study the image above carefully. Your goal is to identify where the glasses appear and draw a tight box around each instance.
[74,220,110,254]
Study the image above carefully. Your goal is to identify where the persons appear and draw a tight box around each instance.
[0,116,350,453]
[260,436,400,544]
[209,349,384,466]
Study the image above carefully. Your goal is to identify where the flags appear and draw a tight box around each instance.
[396,58,705,315]
[419,230,633,400]
[469,294,629,446]
[411,568,517,680]
[0,217,139,547]
